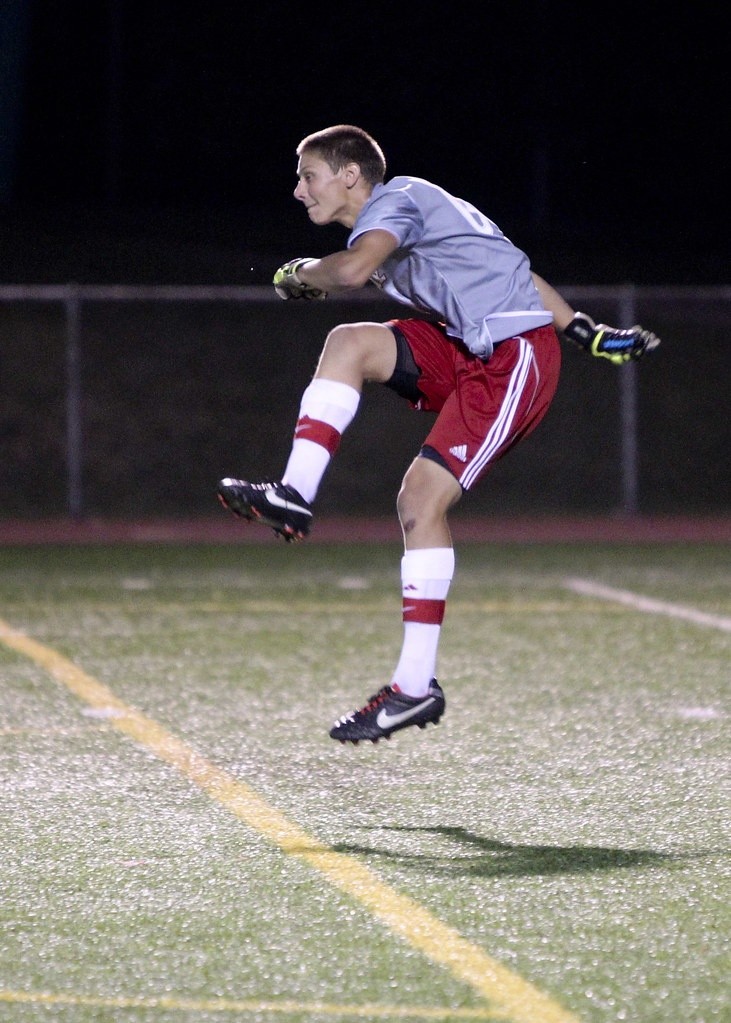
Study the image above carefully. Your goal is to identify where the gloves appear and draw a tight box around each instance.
[561,310,661,365]
[273,258,326,301]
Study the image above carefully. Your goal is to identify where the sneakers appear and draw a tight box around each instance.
[216,477,313,543]
[328,677,447,744]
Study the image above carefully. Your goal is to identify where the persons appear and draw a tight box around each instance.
[216,126,659,746]
